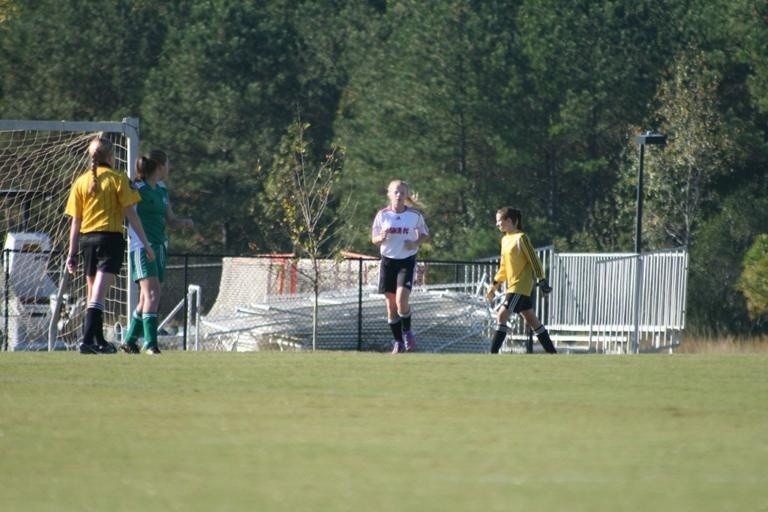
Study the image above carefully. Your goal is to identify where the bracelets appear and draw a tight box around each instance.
[67,253,77,257]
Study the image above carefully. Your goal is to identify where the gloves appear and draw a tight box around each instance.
[536,279,553,295]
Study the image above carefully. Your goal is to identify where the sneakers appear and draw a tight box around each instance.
[404,330,417,352]
[117,343,140,355]
[98,341,118,354]
[392,341,405,354]
[140,344,163,357]
[78,342,101,355]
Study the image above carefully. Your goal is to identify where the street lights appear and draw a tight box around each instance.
[631,130,666,352]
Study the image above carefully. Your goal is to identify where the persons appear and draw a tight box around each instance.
[486,208,558,354]
[63,138,156,357]
[371,180,430,355]
[118,150,194,356]
